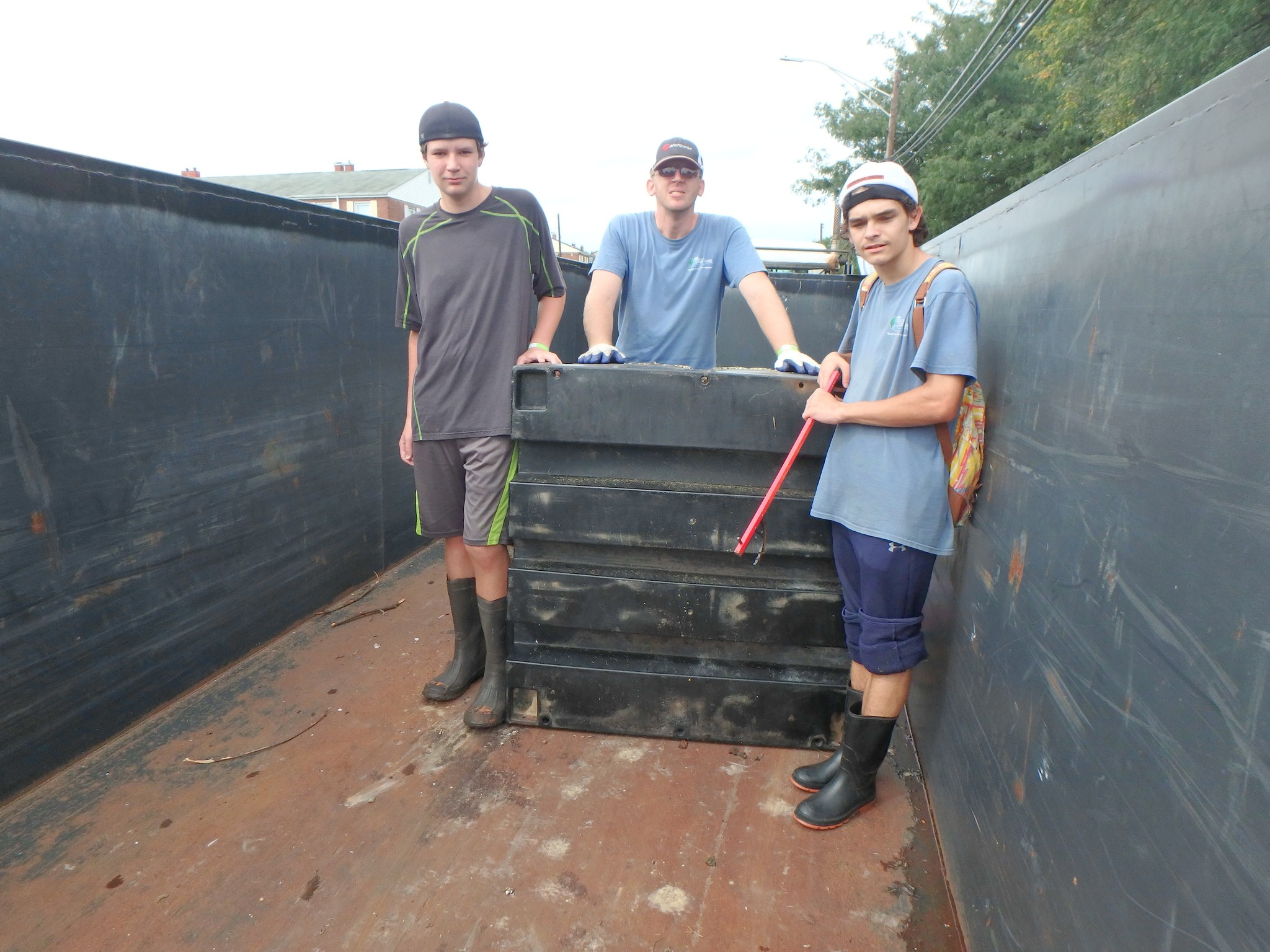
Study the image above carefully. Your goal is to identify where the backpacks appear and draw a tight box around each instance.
[859,263,985,528]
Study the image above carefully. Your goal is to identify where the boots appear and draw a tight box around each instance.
[463,594,507,728]
[793,702,900,831]
[791,681,867,792]
[422,573,487,702]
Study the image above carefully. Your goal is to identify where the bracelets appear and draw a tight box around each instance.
[528,342,549,352]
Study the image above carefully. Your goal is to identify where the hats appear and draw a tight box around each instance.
[652,137,703,173]
[420,102,485,145]
[838,162,928,246]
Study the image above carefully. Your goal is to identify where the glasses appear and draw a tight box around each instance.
[651,167,700,179]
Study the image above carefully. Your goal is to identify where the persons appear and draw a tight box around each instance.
[394,101,562,728]
[578,139,820,376]
[791,158,980,830]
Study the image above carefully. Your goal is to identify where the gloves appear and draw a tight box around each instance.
[576,344,628,364]
[772,343,821,376]
